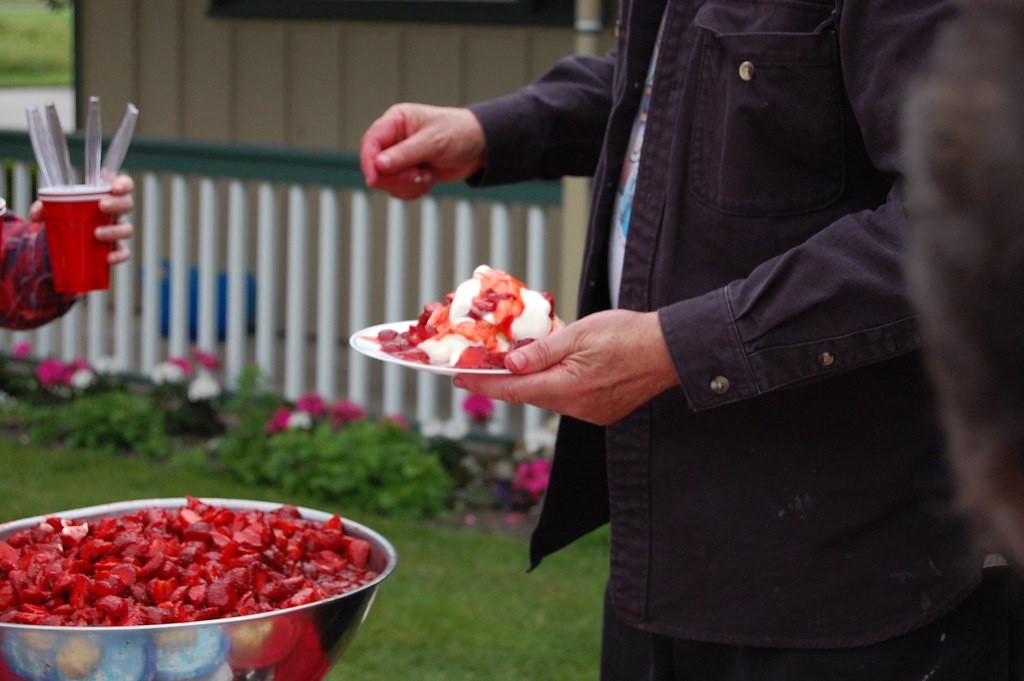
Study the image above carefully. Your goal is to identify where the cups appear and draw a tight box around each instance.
[0,197,6,266]
[37,184,111,293]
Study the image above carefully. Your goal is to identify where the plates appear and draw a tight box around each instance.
[349,319,513,375]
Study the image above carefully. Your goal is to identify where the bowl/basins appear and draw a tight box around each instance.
[1,499,396,680]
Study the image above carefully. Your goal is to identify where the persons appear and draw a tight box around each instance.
[1,173,133,329]
[358,1,1022,681]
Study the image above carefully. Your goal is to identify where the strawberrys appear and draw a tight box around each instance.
[0,496,381,628]
